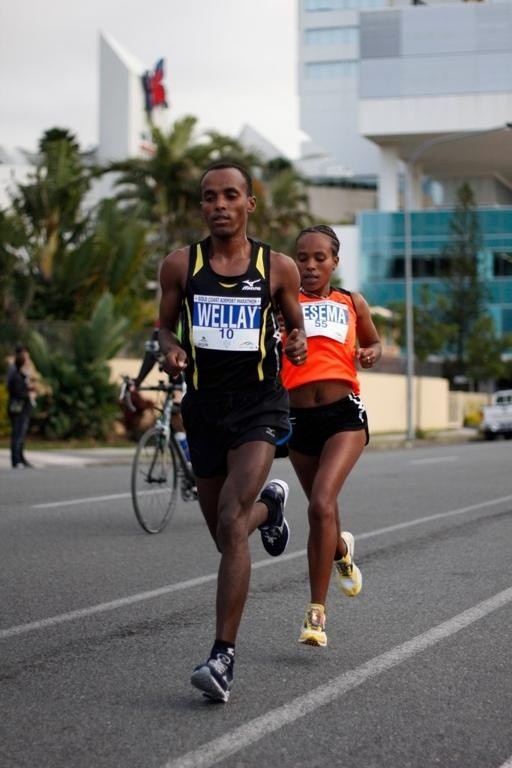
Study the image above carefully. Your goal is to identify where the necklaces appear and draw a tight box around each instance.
[301,287,329,300]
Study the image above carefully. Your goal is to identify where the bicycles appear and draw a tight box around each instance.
[115,371,200,537]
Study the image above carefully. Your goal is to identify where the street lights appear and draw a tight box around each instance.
[404,122,511,443]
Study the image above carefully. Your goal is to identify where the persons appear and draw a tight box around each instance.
[125,318,193,474]
[7,355,34,469]
[277,226,382,647]
[157,159,288,706]
[4,344,46,409]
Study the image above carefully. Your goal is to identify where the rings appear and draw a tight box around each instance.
[367,355,370,359]
[297,355,301,360]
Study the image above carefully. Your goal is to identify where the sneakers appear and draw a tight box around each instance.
[333,530,362,598]
[257,476,290,557]
[189,651,237,705]
[298,602,328,648]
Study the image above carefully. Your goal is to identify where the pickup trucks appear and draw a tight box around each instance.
[479,388,512,440]
